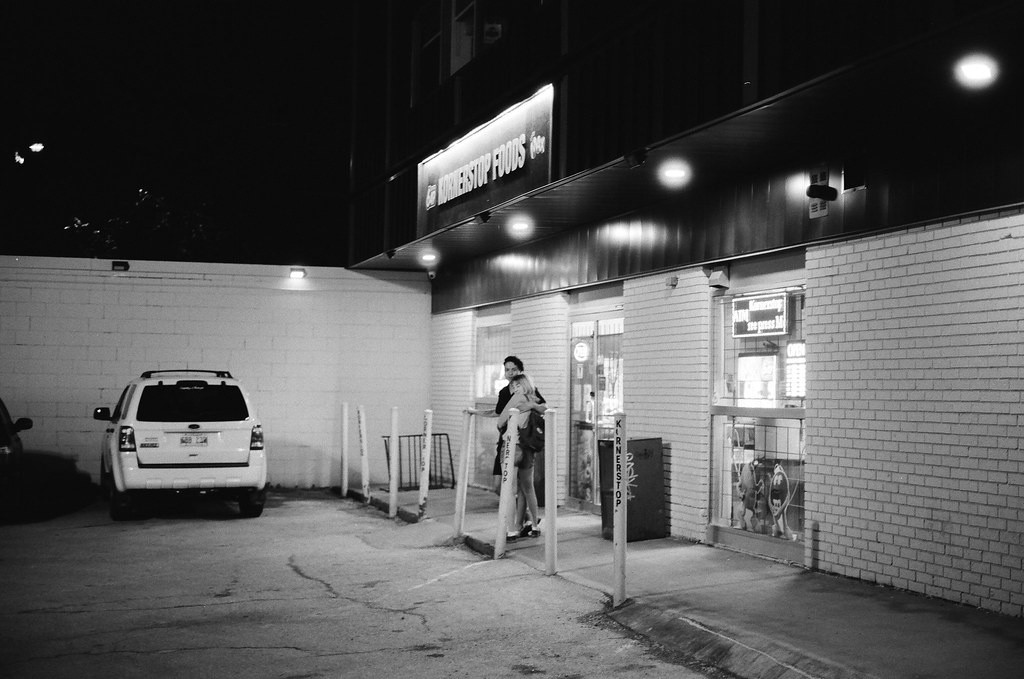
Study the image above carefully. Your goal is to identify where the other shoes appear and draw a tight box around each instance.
[506,536,517,542]
[532,530,540,537]
[521,517,541,536]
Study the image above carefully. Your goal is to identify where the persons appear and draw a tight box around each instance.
[492,356,549,543]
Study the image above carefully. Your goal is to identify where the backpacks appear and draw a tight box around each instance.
[517,394,545,452]
